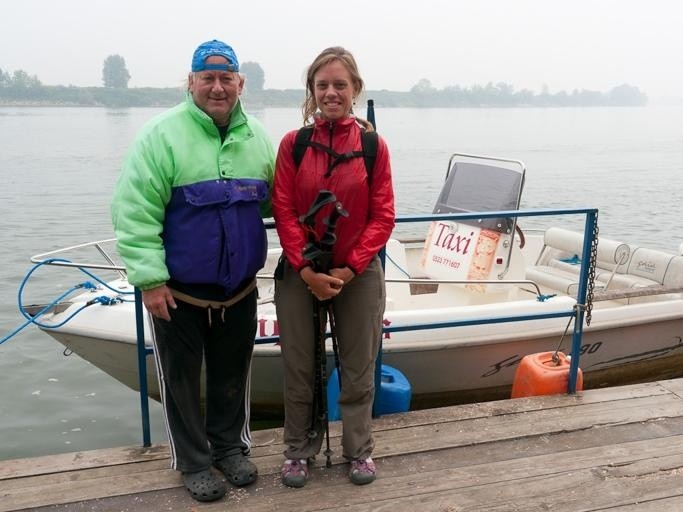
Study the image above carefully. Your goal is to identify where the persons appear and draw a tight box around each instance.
[274,46,394,488]
[112,40,273,502]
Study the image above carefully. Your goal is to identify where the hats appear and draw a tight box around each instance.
[191,37,241,74]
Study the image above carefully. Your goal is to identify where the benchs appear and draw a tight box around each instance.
[519,228,630,297]
[548,232,677,291]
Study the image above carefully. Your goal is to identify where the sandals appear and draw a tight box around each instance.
[349,456,377,486]
[280,455,310,487]
[212,453,260,485]
[181,468,226,503]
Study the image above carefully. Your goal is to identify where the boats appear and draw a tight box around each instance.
[16,151,683,420]
